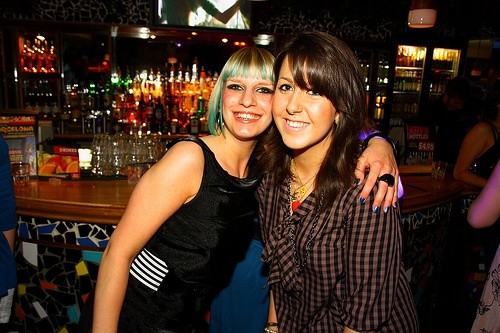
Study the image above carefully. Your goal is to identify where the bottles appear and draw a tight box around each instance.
[392,97,417,114]
[66,60,218,135]
[25,83,60,117]
[394,47,456,93]
[92,135,167,177]
[374,96,387,123]
[20,37,58,73]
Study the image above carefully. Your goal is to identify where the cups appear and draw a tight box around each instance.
[11,162,30,187]
[431,160,447,182]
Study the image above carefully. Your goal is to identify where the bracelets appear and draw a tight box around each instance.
[264,327,277,333]
[266,321,278,327]
[362,132,397,158]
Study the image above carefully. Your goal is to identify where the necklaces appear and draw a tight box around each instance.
[287,160,321,211]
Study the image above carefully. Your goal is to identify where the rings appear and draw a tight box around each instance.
[379,174,395,187]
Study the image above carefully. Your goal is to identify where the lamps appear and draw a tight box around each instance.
[408,0,437,28]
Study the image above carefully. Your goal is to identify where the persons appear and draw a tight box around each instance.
[255,21,424,333]
[0,120,21,333]
[466,157,500,333]
[89,36,401,333]
[451,103,500,285]
